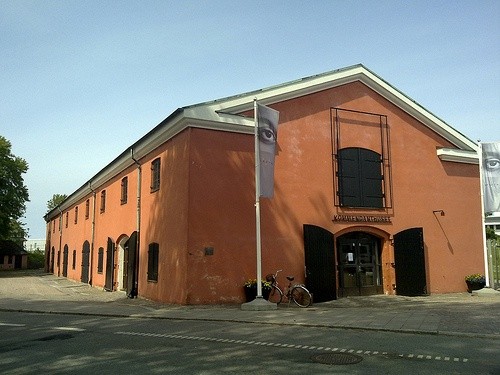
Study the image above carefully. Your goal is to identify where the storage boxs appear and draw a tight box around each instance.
[244,288,272,302]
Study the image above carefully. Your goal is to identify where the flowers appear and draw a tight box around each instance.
[464,273,485,283]
[244,278,273,290]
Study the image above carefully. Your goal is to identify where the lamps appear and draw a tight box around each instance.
[433,209,444,216]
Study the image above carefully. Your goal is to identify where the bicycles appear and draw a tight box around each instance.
[266,270,312,308]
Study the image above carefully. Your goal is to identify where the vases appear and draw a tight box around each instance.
[466,281,485,291]
